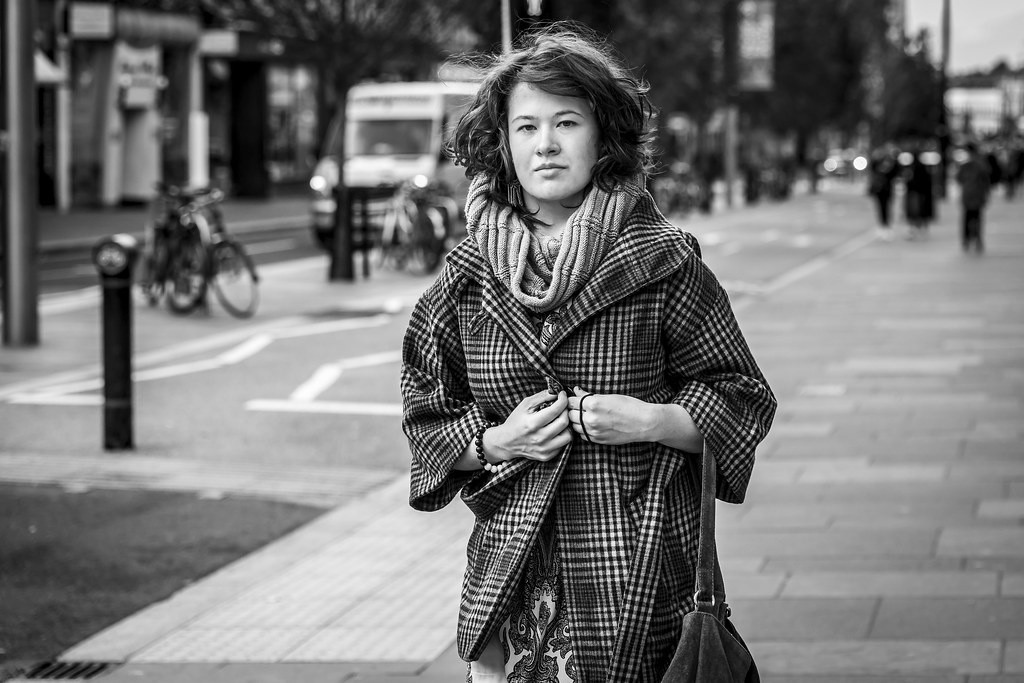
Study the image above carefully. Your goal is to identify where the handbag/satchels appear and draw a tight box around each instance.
[659,436,764,681]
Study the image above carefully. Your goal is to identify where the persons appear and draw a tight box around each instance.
[690,118,1023,250]
[396,29,780,681]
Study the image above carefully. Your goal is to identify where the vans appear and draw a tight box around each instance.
[309,79,481,252]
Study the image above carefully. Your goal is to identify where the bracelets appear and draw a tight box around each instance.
[475,421,512,473]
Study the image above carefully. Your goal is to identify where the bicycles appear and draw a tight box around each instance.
[139,184,260,318]
[369,174,454,271]
